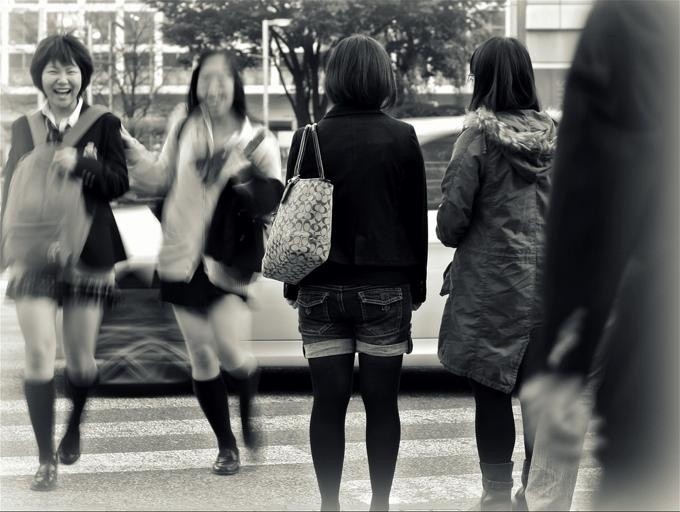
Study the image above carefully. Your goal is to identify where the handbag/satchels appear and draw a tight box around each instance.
[262,173,334,285]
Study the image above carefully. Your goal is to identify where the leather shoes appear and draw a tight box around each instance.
[57,440,80,464]
[30,454,59,491]
[213,449,238,475]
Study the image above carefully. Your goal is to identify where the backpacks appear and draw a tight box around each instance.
[3,105,110,267]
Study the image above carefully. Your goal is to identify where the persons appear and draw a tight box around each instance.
[120,50,283,475]
[527,1,679,510]
[0,30,129,491]
[283,34,428,512]
[436,39,557,511]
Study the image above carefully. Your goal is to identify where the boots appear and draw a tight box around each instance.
[513,459,532,512]
[469,461,514,512]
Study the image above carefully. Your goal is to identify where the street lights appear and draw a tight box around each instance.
[262,18,291,126]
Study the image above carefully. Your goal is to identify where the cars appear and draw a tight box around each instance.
[96,113,466,386]
